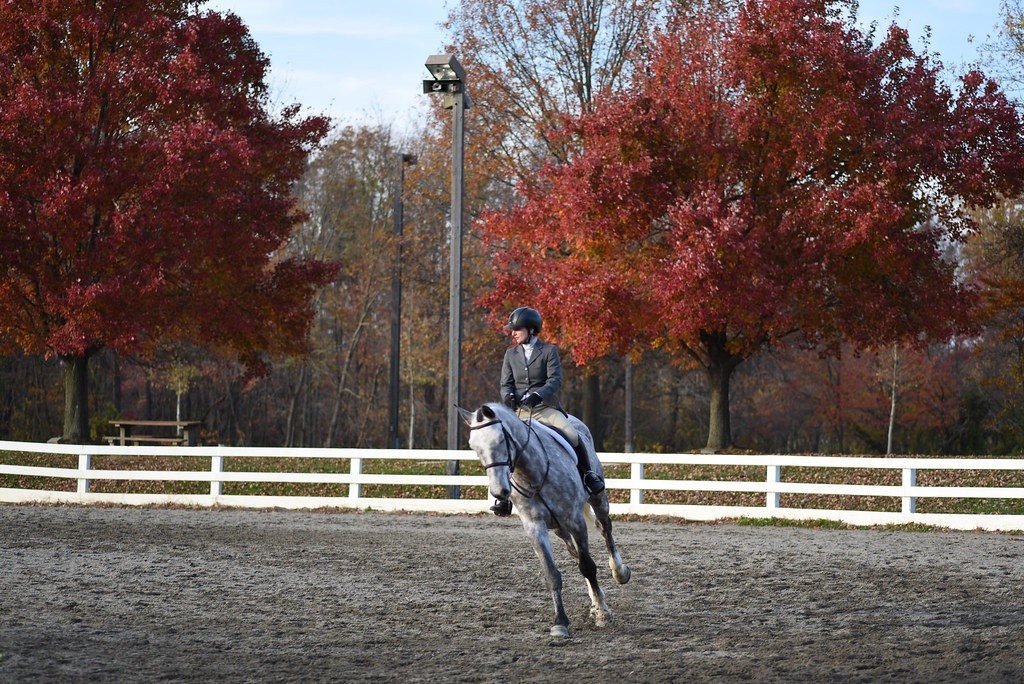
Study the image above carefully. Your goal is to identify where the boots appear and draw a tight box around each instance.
[490,500,513,515]
[571,434,605,495]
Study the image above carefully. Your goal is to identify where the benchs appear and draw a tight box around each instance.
[103,435,203,447]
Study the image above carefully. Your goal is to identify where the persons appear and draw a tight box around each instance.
[489,306,604,515]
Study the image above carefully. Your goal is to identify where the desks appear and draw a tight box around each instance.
[108,420,200,447]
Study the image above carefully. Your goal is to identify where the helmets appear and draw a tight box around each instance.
[503,307,543,332]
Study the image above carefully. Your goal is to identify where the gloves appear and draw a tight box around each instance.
[523,393,542,408]
[504,394,517,407]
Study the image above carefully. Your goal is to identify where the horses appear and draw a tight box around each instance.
[452,401,632,648]
